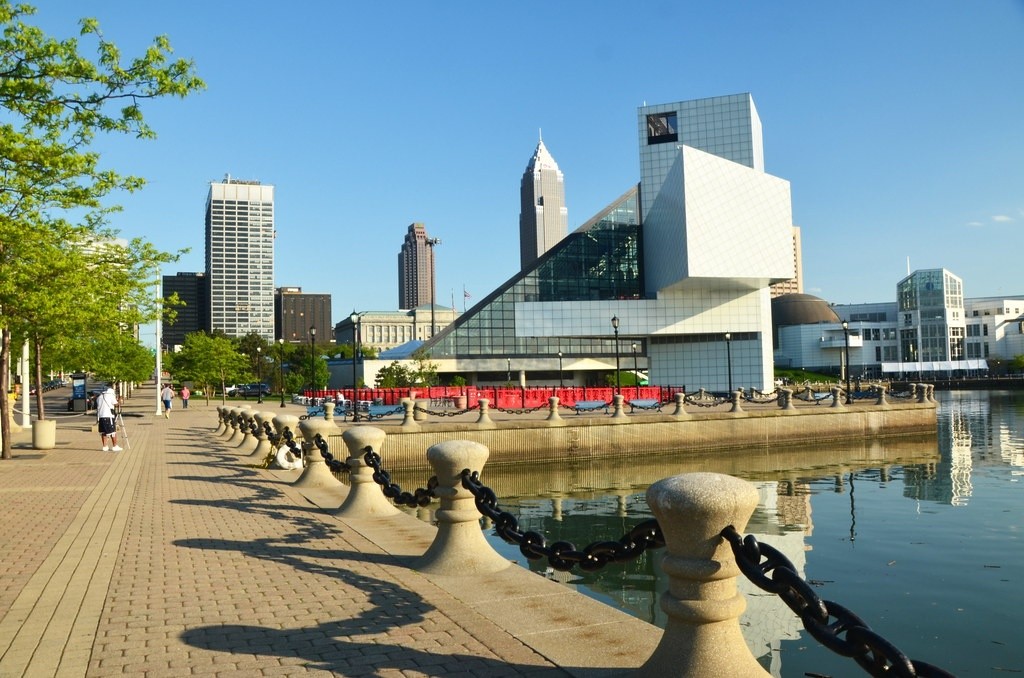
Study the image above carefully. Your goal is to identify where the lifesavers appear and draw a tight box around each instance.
[278,443,303,470]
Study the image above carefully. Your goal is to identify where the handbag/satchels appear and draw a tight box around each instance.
[110,409,117,416]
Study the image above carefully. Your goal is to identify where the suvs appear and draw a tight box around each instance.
[224,383,248,397]
[238,384,271,398]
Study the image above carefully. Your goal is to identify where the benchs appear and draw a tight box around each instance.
[368,406,405,421]
[848,392,879,404]
[575,401,610,415]
[813,392,845,405]
[630,399,662,414]
[307,406,346,422]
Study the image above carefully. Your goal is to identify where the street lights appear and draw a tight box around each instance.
[725,331,733,403]
[309,325,317,407]
[349,310,362,424]
[997,362,1001,374]
[631,343,638,387]
[558,351,563,386]
[508,357,510,388]
[256,345,263,404]
[278,337,287,408]
[610,314,621,396]
[842,318,855,404]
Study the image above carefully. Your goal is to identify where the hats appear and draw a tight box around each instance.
[102,388,116,395]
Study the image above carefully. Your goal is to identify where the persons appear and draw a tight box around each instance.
[181,387,190,409]
[337,391,344,407]
[97,388,124,452]
[161,384,175,419]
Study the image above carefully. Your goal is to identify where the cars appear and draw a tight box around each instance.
[67,388,105,411]
[29,378,67,395]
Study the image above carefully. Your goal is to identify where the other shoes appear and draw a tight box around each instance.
[165,413,170,419]
[103,445,110,452]
[112,445,123,451]
